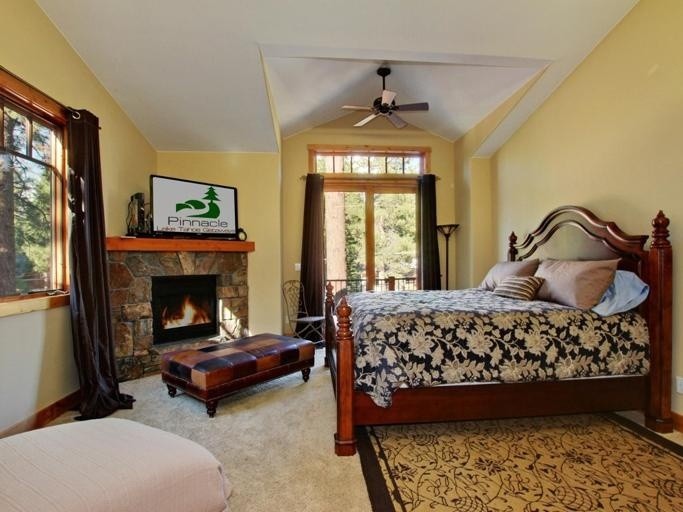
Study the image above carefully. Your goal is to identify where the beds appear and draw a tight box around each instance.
[320,203,672,456]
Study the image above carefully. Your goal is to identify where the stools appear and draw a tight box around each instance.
[0,418,233,512]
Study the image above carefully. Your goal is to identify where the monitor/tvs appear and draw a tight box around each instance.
[149,173,239,241]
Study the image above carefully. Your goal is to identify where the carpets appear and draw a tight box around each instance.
[353,409,683,512]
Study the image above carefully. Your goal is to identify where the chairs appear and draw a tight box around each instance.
[282,279,325,345]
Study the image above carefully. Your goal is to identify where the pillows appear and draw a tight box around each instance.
[478,258,539,289]
[534,257,623,311]
[590,268,650,317]
[493,274,545,301]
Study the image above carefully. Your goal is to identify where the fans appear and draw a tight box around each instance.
[341,67,429,129]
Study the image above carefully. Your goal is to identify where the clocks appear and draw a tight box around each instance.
[237,228,247,240]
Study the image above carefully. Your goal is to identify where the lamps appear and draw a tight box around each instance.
[436,224,459,290]
